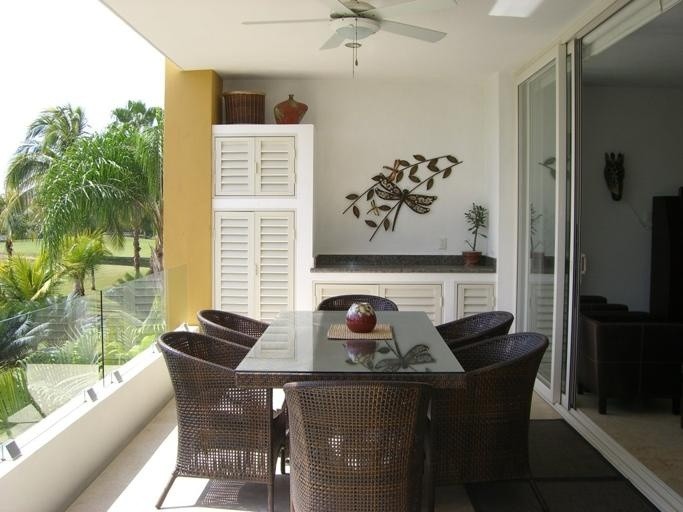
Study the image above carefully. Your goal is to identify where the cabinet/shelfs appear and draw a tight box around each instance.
[451,281,498,322]
[213,210,298,360]
[649,195,681,318]
[213,133,296,197]
[315,281,444,328]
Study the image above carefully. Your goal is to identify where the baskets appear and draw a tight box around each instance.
[221,89,266,125]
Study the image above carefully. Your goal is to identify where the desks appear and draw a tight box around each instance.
[234,311,469,467]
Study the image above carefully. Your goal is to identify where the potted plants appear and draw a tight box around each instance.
[457,201,488,266]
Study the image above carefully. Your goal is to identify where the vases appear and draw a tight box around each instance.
[273,93,309,125]
[346,302,377,333]
[345,338,378,366]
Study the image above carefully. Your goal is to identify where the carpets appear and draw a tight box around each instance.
[460,418,661,511]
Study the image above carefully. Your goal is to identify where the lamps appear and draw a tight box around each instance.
[328,17,379,77]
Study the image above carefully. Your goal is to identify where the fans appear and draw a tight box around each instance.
[240,0,459,54]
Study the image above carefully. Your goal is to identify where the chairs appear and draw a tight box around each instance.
[196,309,273,347]
[282,380,435,511]
[434,310,513,350]
[573,291,682,420]
[421,332,551,511]
[156,331,288,511]
[318,293,398,312]
